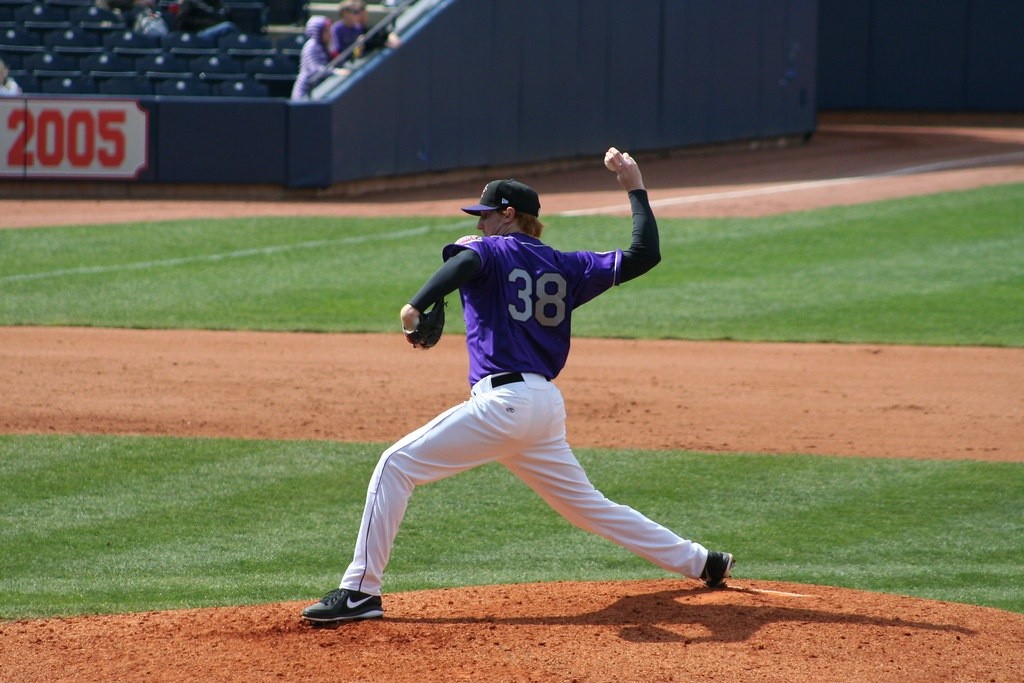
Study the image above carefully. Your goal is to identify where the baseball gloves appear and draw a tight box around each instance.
[400,295,451,351]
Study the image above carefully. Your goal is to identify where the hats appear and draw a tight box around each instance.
[461,179,540,216]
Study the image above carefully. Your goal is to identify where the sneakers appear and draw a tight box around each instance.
[700,550,736,587]
[302,589,383,622]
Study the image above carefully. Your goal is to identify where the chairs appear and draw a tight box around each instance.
[0,0,306,99]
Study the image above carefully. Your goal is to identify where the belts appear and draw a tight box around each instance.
[472,372,551,397]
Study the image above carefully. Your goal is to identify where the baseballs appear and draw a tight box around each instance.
[603,156,622,172]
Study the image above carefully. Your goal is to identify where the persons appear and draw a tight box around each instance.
[292,0,401,99]
[300,144,737,623]
[0,61,22,96]
[94,1,228,34]
